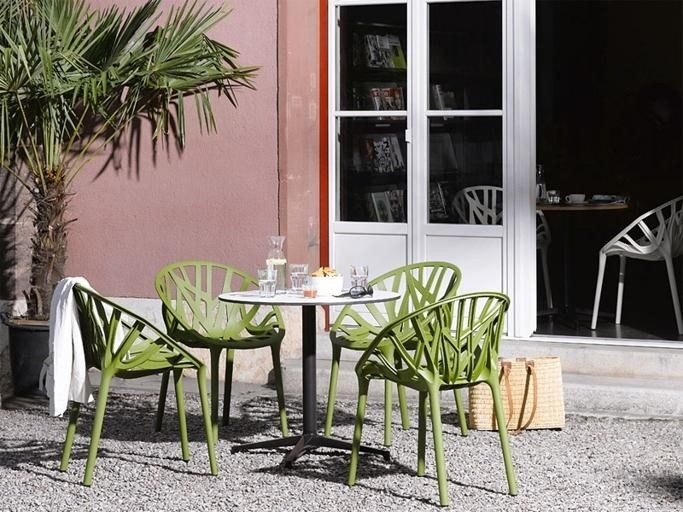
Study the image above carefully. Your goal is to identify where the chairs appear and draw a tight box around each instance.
[592,198,683,335]
[153,260,289,443]
[347,291,517,505]
[60,280,217,485]
[451,186,553,312]
[322,262,467,446]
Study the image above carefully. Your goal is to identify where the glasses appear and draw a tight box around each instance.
[336,285,373,297]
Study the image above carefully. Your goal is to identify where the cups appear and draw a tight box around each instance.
[288,263,310,292]
[547,190,561,204]
[565,193,586,202]
[257,269,278,298]
[349,264,369,292]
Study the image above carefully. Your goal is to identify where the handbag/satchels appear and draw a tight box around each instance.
[467,355,565,431]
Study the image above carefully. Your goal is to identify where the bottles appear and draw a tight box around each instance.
[265,235,288,295]
[536,164,547,204]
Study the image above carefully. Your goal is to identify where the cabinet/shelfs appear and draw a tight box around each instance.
[340,19,466,224]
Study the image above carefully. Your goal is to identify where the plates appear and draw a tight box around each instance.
[567,200,589,207]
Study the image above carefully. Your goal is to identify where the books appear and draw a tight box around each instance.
[363,29,459,222]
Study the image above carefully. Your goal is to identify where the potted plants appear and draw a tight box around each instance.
[0,0,260,399]
